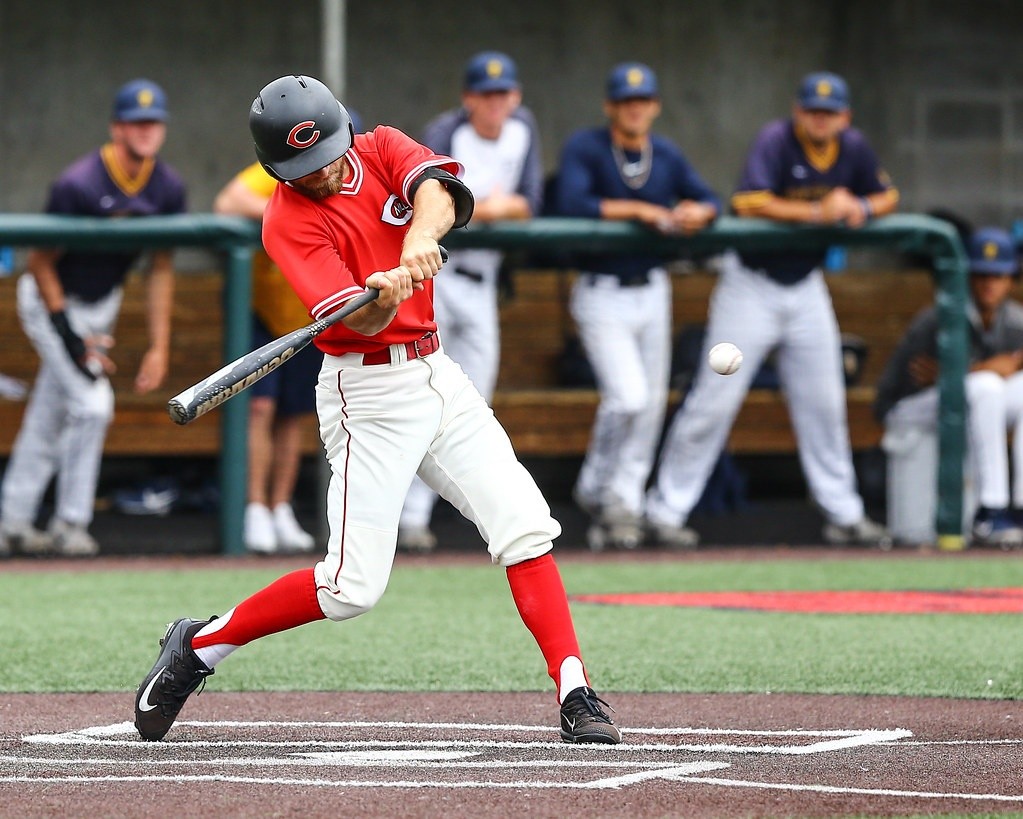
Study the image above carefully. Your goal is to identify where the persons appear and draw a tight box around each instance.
[2,82,188,558]
[560,60,733,547]
[213,107,364,552]
[394,51,550,552]
[646,69,900,550]
[135,76,621,745]
[871,225,1023,551]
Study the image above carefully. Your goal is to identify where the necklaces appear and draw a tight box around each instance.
[612,131,652,189]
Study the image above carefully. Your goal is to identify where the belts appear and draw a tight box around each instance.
[456,267,483,283]
[362,332,438,366]
[588,270,650,288]
[740,251,824,286]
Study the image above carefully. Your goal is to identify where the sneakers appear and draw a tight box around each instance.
[0,520,97,556]
[823,518,886,549]
[242,500,315,555]
[586,513,643,553]
[134,615,219,742]
[648,522,699,551]
[398,527,437,553]
[974,508,1023,547]
[560,686,623,744]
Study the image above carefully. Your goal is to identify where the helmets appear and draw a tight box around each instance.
[112,80,172,120]
[248,75,355,183]
[464,51,520,93]
[605,63,659,100]
[966,221,1023,273]
[798,72,851,113]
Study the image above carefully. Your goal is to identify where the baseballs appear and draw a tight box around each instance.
[708,342,743,376]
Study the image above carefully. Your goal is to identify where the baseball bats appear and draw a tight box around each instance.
[166,243,449,427]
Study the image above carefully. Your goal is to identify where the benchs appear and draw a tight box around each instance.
[0,255,1023,457]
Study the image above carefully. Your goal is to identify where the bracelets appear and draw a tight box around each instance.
[859,196,875,223]
